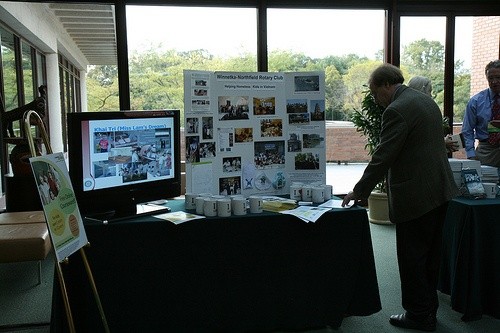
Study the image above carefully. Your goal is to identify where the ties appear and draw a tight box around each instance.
[492,94,500,120]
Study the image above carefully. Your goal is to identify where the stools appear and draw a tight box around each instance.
[0,210,53,283]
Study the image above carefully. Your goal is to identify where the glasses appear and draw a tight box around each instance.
[370,89,379,99]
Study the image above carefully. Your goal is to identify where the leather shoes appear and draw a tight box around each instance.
[389,313,437,332]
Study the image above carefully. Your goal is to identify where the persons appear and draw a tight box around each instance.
[408,76,460,153]
[342,62,458,332]
[98,78,320,197]
[39,171,60,206]
[461,60,500,177]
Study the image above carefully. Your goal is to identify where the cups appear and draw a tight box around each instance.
[249,196,264,214]
[184,193,198,210]
[289,181,333,203]
[196,193,247,217]
[483,182,497,198]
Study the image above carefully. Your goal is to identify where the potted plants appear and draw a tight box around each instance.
[352,85,395,226]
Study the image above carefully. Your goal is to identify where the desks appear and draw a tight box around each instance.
[45,195,383,333]
[434,195,500,323]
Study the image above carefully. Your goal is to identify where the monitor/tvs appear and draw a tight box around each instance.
[67,109,182,224]
